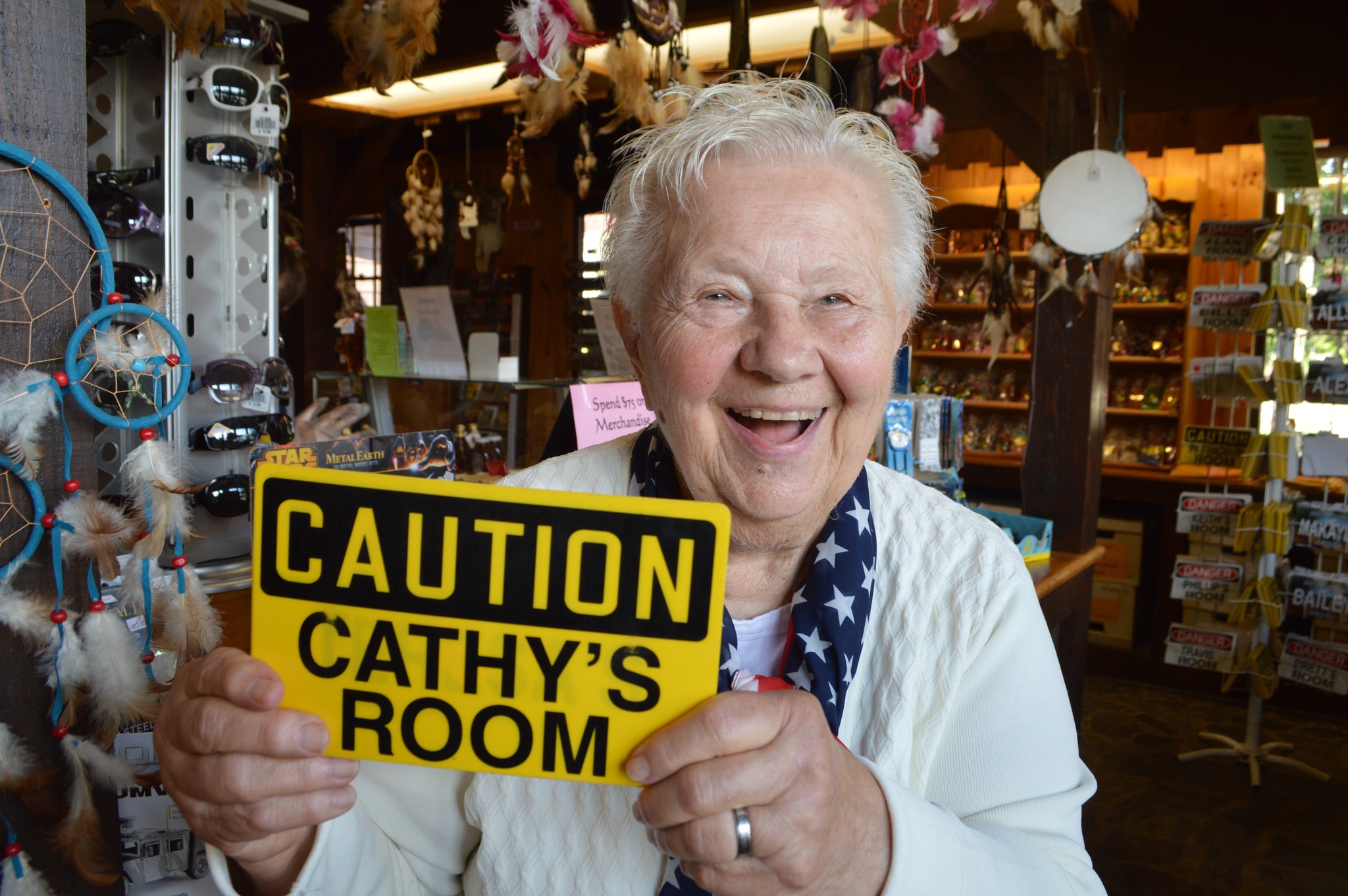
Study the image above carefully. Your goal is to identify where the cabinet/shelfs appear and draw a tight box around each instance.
[912,199,1188,470]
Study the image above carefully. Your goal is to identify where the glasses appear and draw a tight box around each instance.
[188,356,294,403]
[88,167,160,195]
[88,190,164,239]
[188,474,250,518]
[187,134,283,185]
[187,63,292,129]
[86,18,165,61]
[200,11,286,64]
[92,321,142,346]
[94,374,166,408]
[90,261,163,304]
[189,414,294,451]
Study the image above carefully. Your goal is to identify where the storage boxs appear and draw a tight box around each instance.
[1087,518,1143,649]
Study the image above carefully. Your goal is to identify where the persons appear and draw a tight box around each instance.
[155,69,1107,896]
[278,211,371,441]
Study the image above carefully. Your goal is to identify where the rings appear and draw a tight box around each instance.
[733,806,753,856]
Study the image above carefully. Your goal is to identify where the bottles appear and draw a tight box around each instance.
[453,423,505,476]
